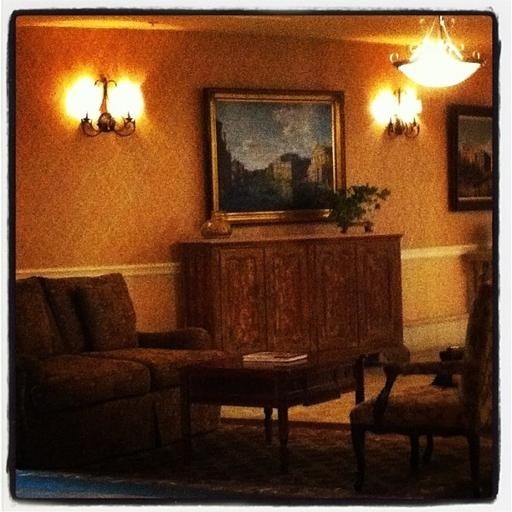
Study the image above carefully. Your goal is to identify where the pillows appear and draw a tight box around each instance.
[69,281,139,351]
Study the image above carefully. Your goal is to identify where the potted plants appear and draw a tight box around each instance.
[318,181,392,234]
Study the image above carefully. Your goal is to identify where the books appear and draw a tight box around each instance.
[243,351,308,361]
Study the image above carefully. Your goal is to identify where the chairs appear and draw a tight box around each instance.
[348,280,497,496]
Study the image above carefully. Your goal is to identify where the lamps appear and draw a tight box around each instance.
[388,16,488,91]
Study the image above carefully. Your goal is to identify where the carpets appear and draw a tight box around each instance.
[13,417,497,503]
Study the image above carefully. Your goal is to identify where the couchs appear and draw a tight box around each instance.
[11,271,225,470]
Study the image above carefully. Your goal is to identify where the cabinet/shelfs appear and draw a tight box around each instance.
[174,232,410,407]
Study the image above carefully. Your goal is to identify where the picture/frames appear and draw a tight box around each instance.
[203,85,348,225]
[444,102,496,211]
